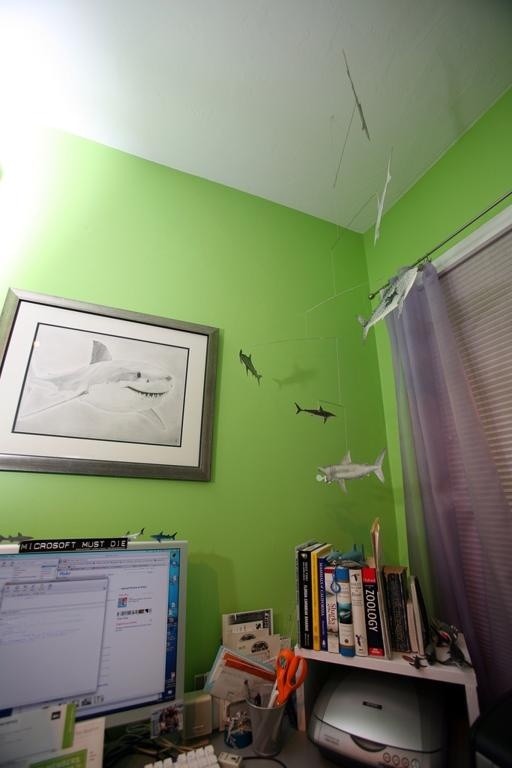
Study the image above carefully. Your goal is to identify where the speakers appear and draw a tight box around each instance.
[179,690,214,739]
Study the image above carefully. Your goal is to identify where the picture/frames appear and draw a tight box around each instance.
[0,288,220,484]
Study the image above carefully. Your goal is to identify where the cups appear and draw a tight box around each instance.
[244,683,290,758]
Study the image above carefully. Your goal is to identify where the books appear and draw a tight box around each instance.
[296,518,428,663]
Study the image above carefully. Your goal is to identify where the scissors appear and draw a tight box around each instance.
[255,648,308,751]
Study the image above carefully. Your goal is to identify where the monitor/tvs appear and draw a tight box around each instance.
[0,536,179,768]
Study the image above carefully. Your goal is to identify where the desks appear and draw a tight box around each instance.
[103,717,499,767]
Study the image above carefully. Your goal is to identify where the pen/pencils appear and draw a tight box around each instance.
[242,665,285,754]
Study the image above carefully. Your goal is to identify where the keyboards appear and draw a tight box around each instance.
[142,743,221,768]
